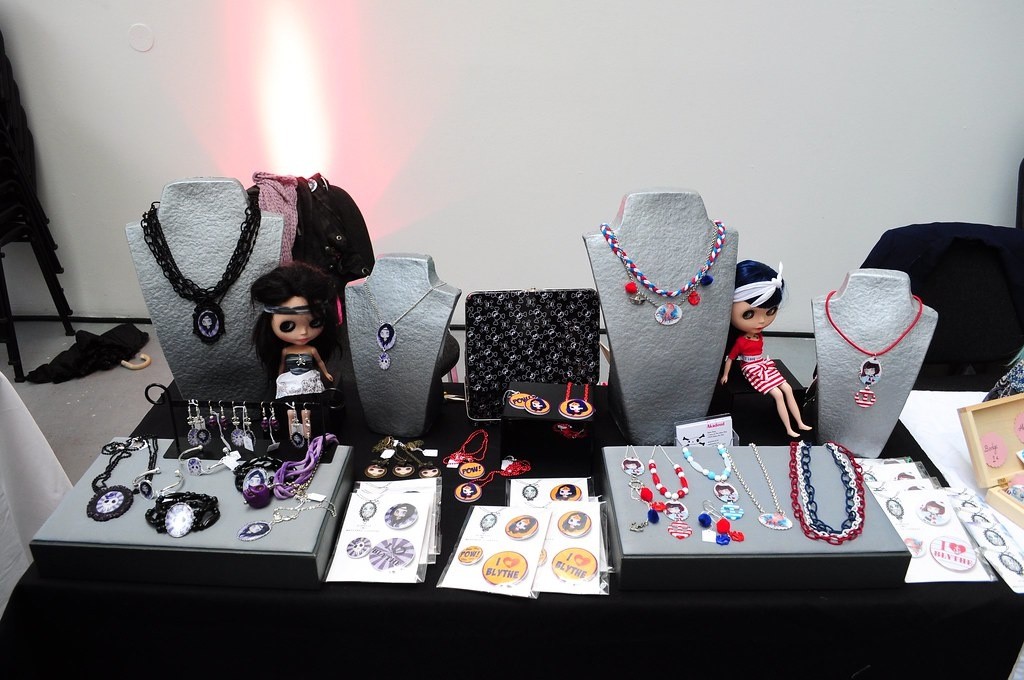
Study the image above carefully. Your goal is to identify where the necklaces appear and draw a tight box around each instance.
[140,186,264,346]
[558,381,596,420]
[364,274,447,370]
[145,491,221,538]
[453,456,531,502]
[825,289,923,409]
[621,445,659,532]
[682,444,745,521]
[600,219,726,325]
[789,440,865,545]
[87,433,160,521]
[442,429,489,480]
[232,433,339,541]
[722,443,792,530]
[649,445,692,539]
[364,436,442,479]
[552,421,590,439]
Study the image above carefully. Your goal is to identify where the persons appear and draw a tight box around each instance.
[251,263,343,437]
[720,260,812,438]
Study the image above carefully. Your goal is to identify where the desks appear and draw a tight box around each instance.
[0,375,1024,680]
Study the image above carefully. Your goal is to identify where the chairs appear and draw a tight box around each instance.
[814,223,1024,395]
[0,28,75,383]
